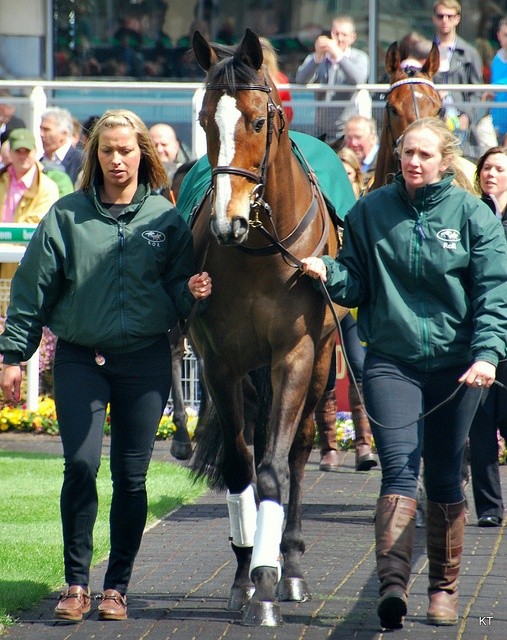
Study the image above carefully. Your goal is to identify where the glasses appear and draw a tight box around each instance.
[434,11,458,21]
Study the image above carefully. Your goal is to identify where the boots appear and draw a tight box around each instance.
[351,404,378,471]
[425,497,465,625]
[315,387,340,473]
[374,494,419,630]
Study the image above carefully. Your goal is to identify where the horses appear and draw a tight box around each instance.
[363,37,480,202]
[186,21,353,631]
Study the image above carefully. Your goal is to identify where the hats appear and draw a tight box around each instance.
[8,128,36,152]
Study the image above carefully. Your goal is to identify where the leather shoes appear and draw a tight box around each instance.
[98,588,129,621]
[477,515,503,528]
[55,583,91,622]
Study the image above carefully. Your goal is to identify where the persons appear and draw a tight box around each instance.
[1,94,27,141]
[473,378,482,388]
[466,145,507,531]
[315,148,379,474]
[429,0,483,144]
[342,114,380,191]
[147,121,180,163]
[39,110,87,190]
[80,114,99,148]
[260,37,293,124]
[0,126,60,225]
[0,108,212,625]
[293,15,371,149]
[480,18,506,145]
[298,116,506,630]
